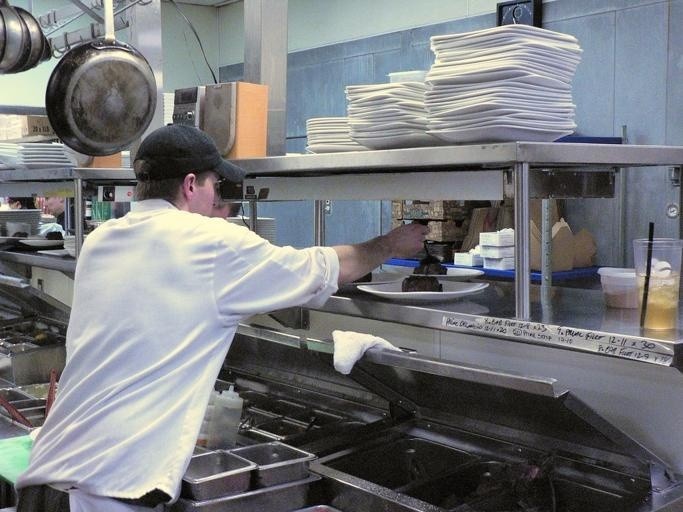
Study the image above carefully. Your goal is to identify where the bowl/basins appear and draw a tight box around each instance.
[597,268,640,309]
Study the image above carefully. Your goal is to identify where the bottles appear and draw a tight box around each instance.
[206,385,244,448]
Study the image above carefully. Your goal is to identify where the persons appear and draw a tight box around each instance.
[0,196,87,235]
[13,123,429,512]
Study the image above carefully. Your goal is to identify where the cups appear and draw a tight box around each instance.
[632,238,682,331]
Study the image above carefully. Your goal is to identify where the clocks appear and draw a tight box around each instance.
[495,0,543,27]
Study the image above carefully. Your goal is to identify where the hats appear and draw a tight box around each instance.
[9,195,37,209]
[132,123,248,184]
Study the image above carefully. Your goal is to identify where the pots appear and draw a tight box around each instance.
[1,0,51,73]
[44,0,158,158]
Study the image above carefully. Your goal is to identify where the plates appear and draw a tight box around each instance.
[63,235,88,259]
[343,82,452,150]
[423,23,585,145]
[304,115,369,151]
[18,239,64,246]
[18,142,77,169]
[0,209,41,237]
[225,214,277,245]
[350,264,491,301]
[0,142,20,167]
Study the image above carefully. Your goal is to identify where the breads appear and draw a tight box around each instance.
[413,255,448,276]
[12,230,30,237]
[46,231,63,240]
[353,272,372,282]
[401,274,443,293]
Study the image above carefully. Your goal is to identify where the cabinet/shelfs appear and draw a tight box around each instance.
[0,141,682,368]
